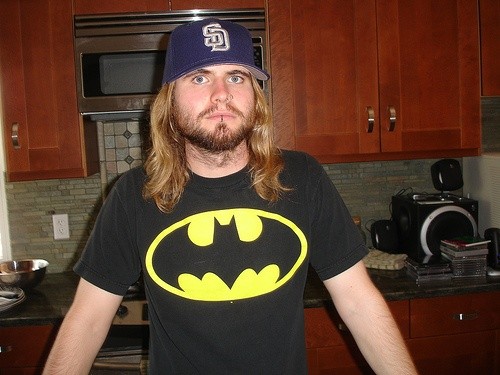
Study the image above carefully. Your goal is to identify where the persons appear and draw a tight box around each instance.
[43,18,418,375]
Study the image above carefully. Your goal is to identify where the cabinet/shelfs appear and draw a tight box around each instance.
[72,0,264,16]
[0,320,62,375]
[266,0,482,164]
[479,0,500,98]
[0,0,103,184]
[303,291,500,375]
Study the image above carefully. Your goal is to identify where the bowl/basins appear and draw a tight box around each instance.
[0,258,49,289]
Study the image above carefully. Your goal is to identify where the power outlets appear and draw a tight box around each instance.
[52,214,71,240]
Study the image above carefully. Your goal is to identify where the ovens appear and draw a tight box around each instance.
[88,353,148,375]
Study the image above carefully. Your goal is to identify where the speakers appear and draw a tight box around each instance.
[370,220,392,253]
[483,228,500,271]
[431,158,464,191]
[391,194,479,268]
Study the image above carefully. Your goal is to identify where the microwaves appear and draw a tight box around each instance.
[74,7,267,121]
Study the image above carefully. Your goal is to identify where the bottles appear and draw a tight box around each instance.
[353,215,367,245]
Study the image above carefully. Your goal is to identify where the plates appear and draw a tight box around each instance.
[0,286,27,313]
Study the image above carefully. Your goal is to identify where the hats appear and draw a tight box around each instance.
[163,18,268,84]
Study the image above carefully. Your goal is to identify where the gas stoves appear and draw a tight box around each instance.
[111,273,152,327]
